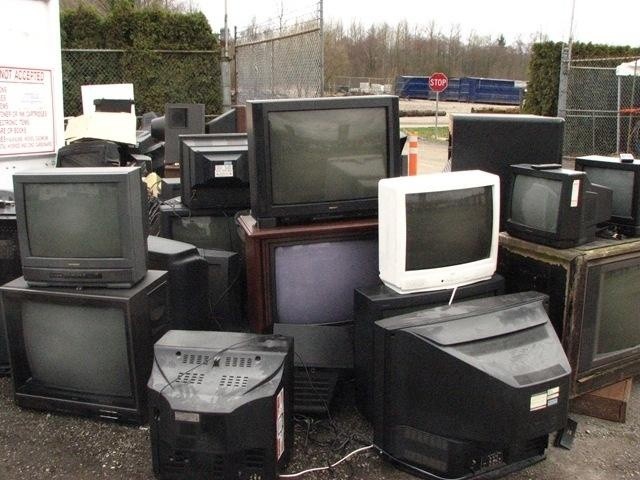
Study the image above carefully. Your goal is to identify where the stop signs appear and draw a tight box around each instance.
[428,73,448,92]
